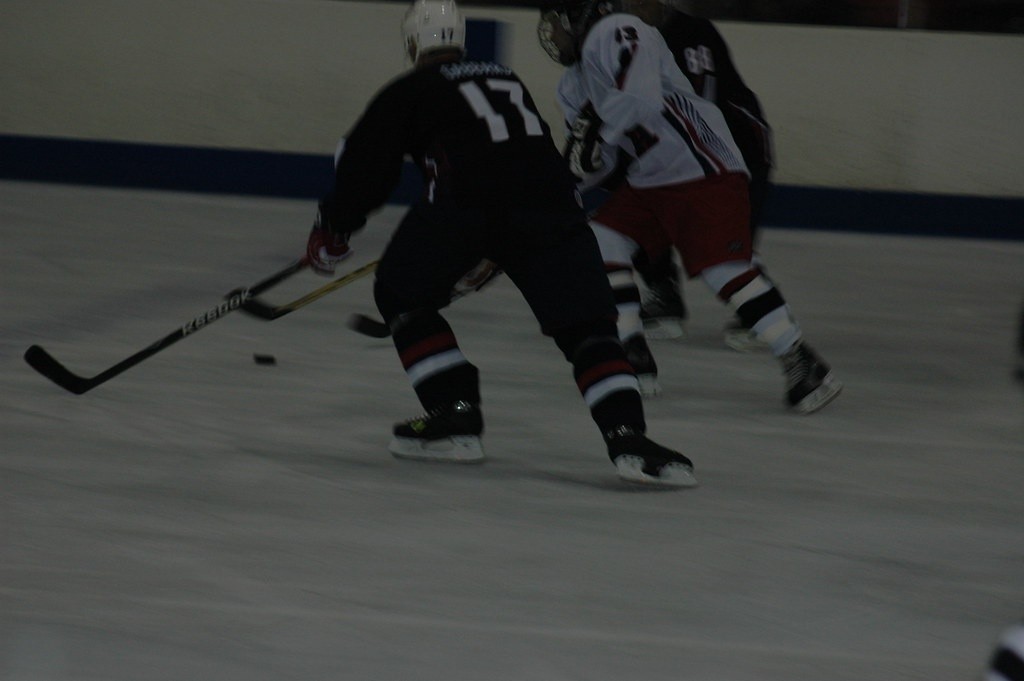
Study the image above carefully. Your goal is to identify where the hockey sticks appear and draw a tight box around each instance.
[223,139,605,341]
[23,248,313,396]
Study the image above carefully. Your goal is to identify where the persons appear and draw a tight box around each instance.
[536,0,845,416]
[611,0,799,356]
[307,0,697,491]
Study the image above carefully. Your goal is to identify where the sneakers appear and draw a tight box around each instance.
[389,400,486,465]
[620,330,663,398]
[605,424,698,487]
[781,335,846,417]
[721,262,804,355]
[630,243,690,340]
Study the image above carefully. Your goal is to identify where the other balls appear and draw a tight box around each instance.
[250,351,277,368]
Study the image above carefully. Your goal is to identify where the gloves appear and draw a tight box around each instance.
[306,207,356,278]
[564,98,607,179]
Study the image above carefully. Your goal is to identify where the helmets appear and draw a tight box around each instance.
[537,0,615,66]
[401,0,466,65]
